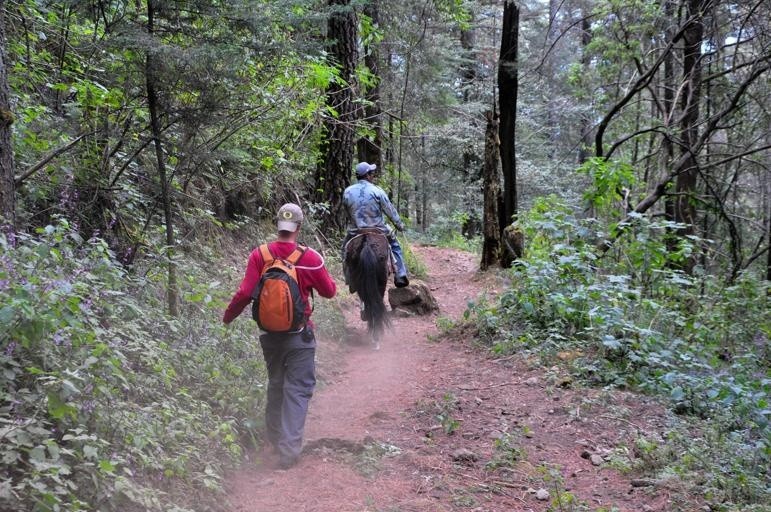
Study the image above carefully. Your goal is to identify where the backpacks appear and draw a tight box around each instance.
[253,245,307,332]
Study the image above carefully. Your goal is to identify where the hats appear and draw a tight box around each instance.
[356,162,376,177]
[277,203,303,232]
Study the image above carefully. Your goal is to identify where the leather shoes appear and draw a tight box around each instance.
[395,276,409,287]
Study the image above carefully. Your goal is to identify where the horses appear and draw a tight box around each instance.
[346,231,396,350]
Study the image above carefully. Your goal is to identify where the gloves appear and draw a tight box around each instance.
[396,222,404,231]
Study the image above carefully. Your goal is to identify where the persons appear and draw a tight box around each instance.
[224,203,336,469]
[341,162,409,288]
